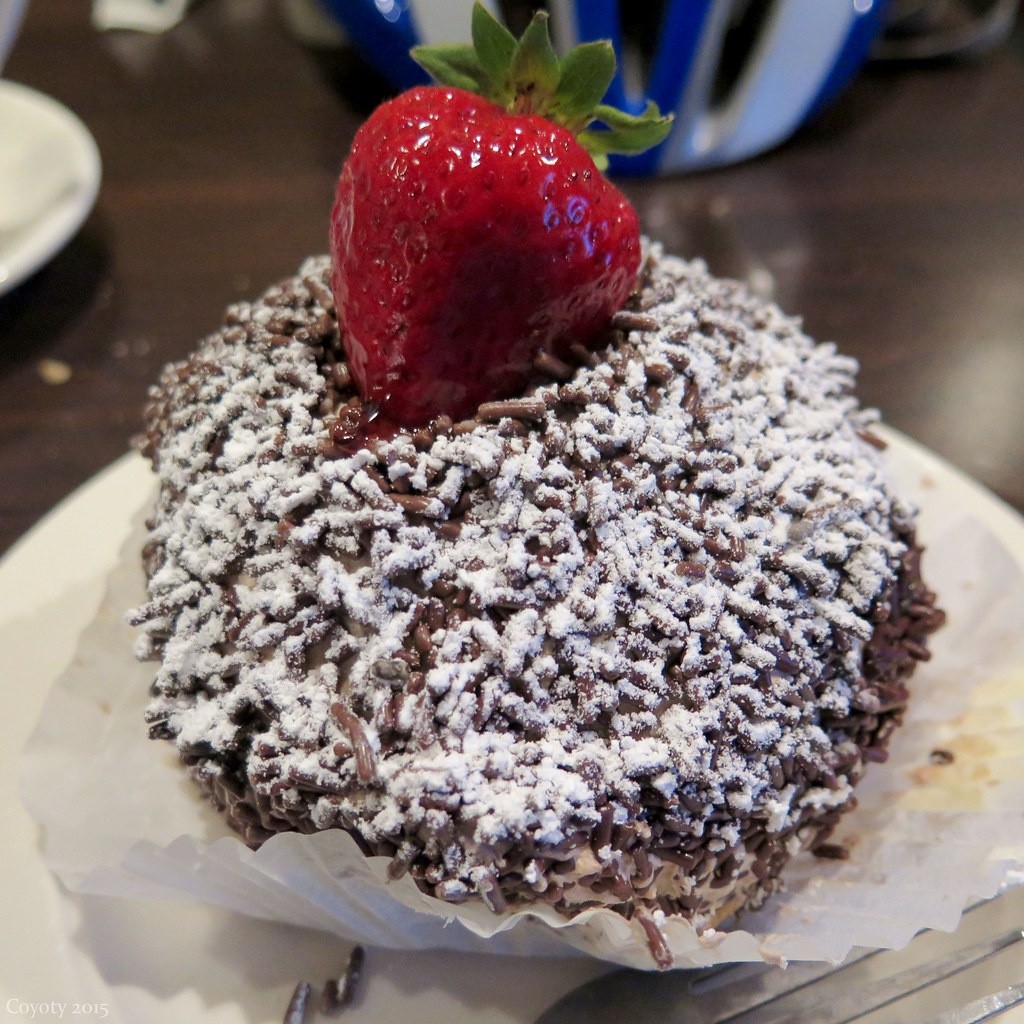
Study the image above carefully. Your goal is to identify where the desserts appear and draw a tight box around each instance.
[140,237,949,974]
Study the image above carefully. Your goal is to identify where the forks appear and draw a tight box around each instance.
[533,887,1022,1024]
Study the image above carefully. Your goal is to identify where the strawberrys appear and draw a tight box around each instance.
[327,0,674,438]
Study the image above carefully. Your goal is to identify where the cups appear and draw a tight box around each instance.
[276,1,886,180]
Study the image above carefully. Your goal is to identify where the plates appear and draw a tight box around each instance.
[0,77,101,302]
[0,412,1023,1024]
